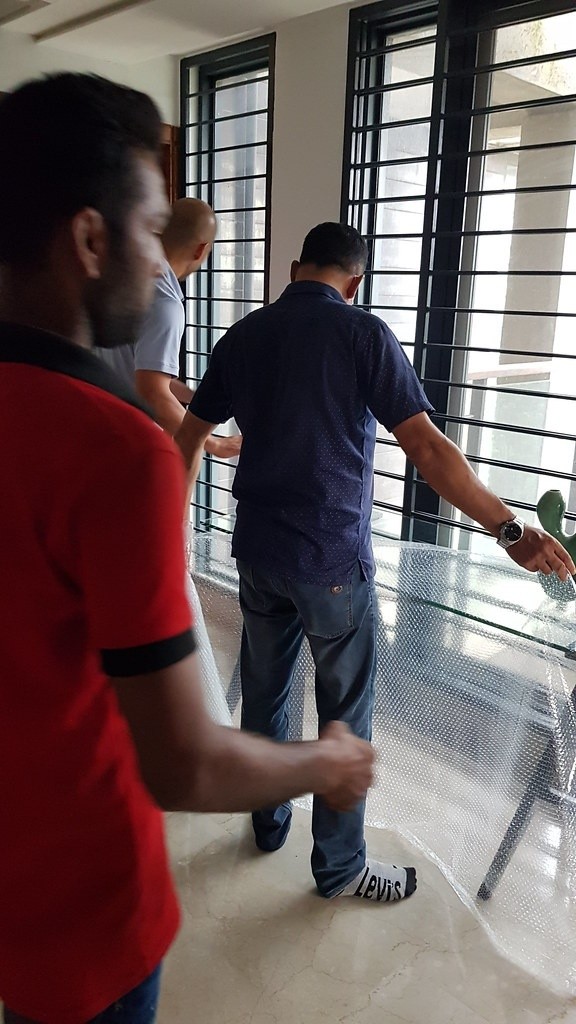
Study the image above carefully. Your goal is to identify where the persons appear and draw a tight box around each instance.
[0,71,376,1024]
[174,222,576,902]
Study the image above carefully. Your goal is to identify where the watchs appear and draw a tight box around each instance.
[496,515,525,549]
